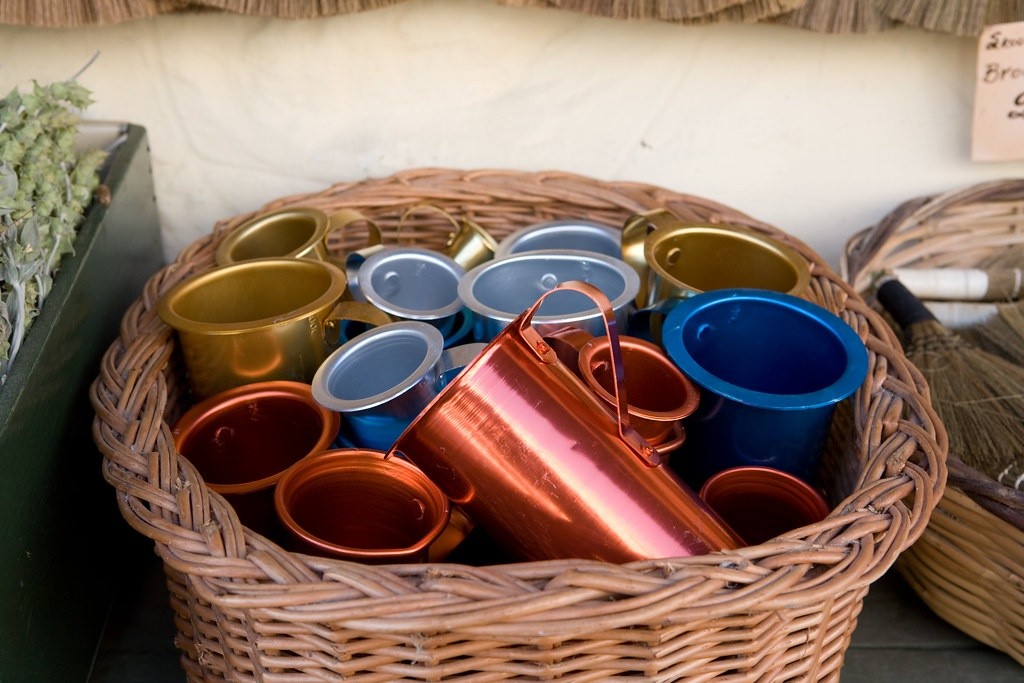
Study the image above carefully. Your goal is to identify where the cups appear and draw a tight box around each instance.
[156,203,871,562]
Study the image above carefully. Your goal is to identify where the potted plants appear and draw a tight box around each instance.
[0,79,166,683]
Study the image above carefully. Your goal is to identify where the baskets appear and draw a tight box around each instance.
[90,166,949,682]
[841,178,1024,666]
